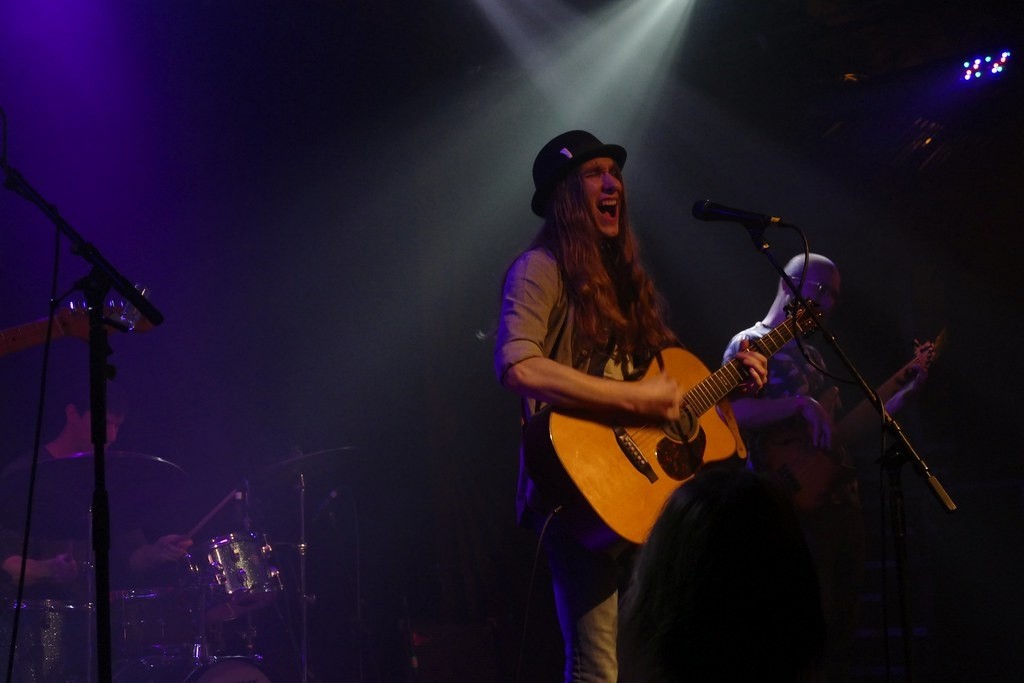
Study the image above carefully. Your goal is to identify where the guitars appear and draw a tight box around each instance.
[757,332,947,517]
[523,299,826,557]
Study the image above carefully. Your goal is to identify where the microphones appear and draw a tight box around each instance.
[692,200,783,226]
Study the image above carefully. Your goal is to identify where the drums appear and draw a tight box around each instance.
[92,588,189,651]
[181,529,280,624]
[108,647,277,683]
[0,597,98,682]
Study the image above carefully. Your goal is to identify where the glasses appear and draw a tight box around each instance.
[792,276,843,301]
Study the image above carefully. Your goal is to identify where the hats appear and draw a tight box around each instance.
[530,129,628,219]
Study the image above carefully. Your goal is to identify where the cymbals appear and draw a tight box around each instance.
[246,446,360,484]
[18,451,192,532]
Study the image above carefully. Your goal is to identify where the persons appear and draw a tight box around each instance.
[0,379,194,589]
[721,253,925,451]
[493,133,769,683]
